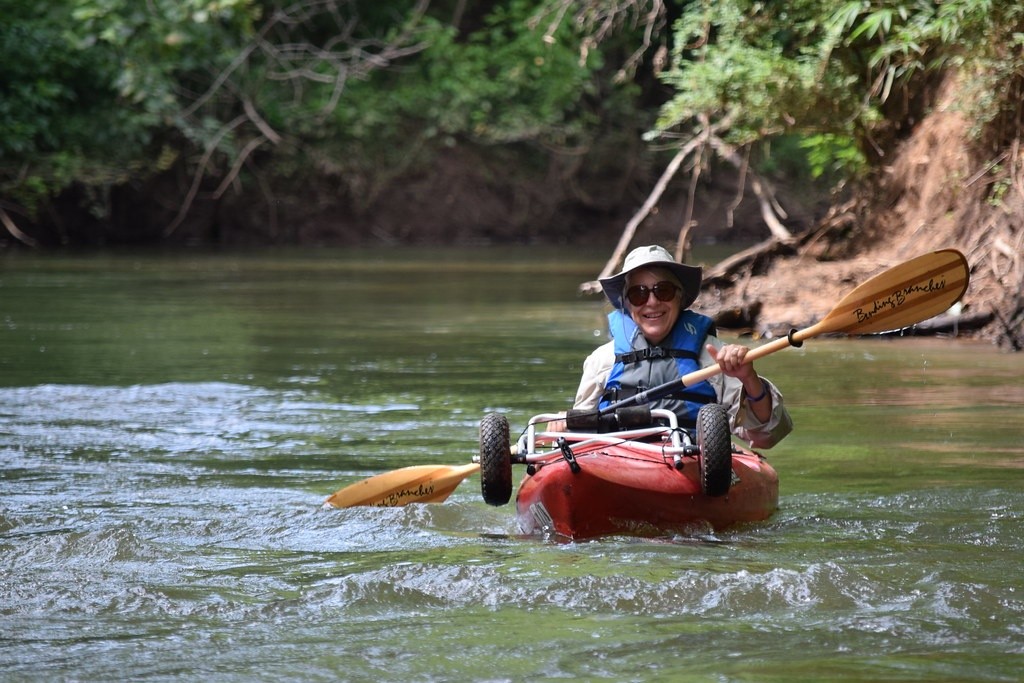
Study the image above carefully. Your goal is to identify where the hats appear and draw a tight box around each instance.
[599,244,703,312]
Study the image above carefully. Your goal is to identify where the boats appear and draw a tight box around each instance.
[516,437,778,544]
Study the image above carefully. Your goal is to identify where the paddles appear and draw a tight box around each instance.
[326,247,973,512]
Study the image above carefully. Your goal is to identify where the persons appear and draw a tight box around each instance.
[545,247,792,452]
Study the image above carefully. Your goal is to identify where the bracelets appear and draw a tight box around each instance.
[742,380,769,401]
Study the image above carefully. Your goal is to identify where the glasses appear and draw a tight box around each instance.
[623,278,684,307]
